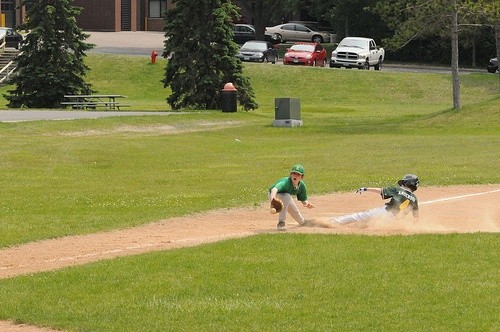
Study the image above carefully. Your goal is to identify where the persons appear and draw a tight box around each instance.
[329,174,419,226]
[269,164,315,231]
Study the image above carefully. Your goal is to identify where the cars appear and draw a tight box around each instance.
[487,56,498,72]
[283,42,326,68]
[235,24,256,40]
[0,27,22,50]
[237,39,278,64]
[265,21,334,42]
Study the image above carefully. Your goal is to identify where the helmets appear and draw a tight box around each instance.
[398,174,419,192]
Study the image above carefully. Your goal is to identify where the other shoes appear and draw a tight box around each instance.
[277,221,286,231]
[301,219,314,226]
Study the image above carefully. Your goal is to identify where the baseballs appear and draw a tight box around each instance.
[271,207,276,214]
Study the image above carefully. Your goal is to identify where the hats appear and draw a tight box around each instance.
[290,165,304,175]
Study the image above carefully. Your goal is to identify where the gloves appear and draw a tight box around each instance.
[355,187,367,195]
[304,202,316,209]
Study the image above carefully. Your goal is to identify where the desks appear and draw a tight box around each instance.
[64,95,126,111]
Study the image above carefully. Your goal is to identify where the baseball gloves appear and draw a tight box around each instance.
[271,197,284,213]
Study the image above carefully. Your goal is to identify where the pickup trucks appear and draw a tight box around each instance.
[331,37,387,72]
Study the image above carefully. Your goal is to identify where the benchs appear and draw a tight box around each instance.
[73,105,131,110]
[60,102,119,109]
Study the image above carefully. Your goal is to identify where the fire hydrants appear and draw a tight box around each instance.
[151,50,157,63]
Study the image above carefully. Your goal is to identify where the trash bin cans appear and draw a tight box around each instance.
[221,82,238,113]
